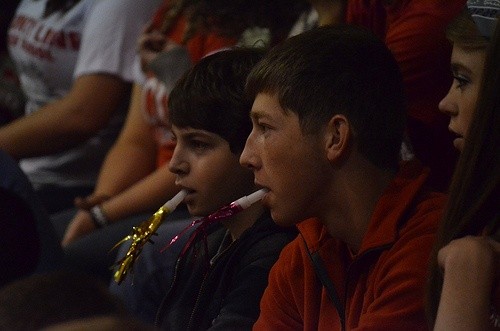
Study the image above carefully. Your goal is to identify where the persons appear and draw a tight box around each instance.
[239,23,449,331]
[0,0,311,331]
[152,48,300,331]
[0,0,162,287]
[301,0,500,331]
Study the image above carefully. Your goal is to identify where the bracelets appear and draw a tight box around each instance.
[88,202,109,231]
